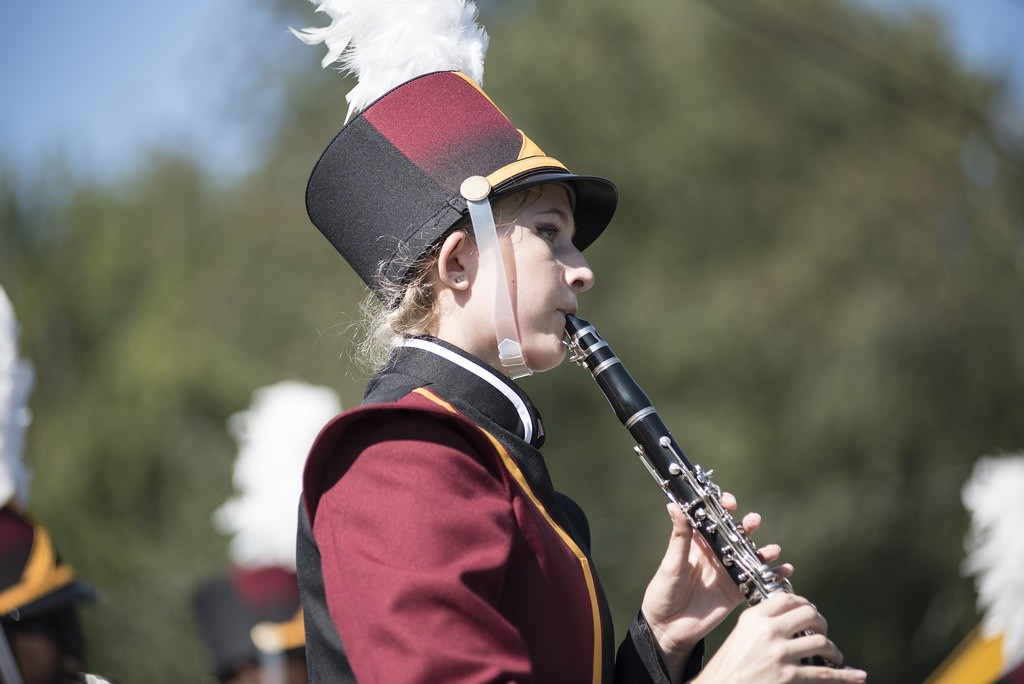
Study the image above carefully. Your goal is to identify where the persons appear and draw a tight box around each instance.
[0,283,368,684]
[294,2,869,684]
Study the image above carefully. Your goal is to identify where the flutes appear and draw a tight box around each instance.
[565,311,839,669]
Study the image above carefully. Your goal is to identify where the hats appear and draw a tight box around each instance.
[289,0,619,309]
[193,382,308,678]
[0,286,96,628]
